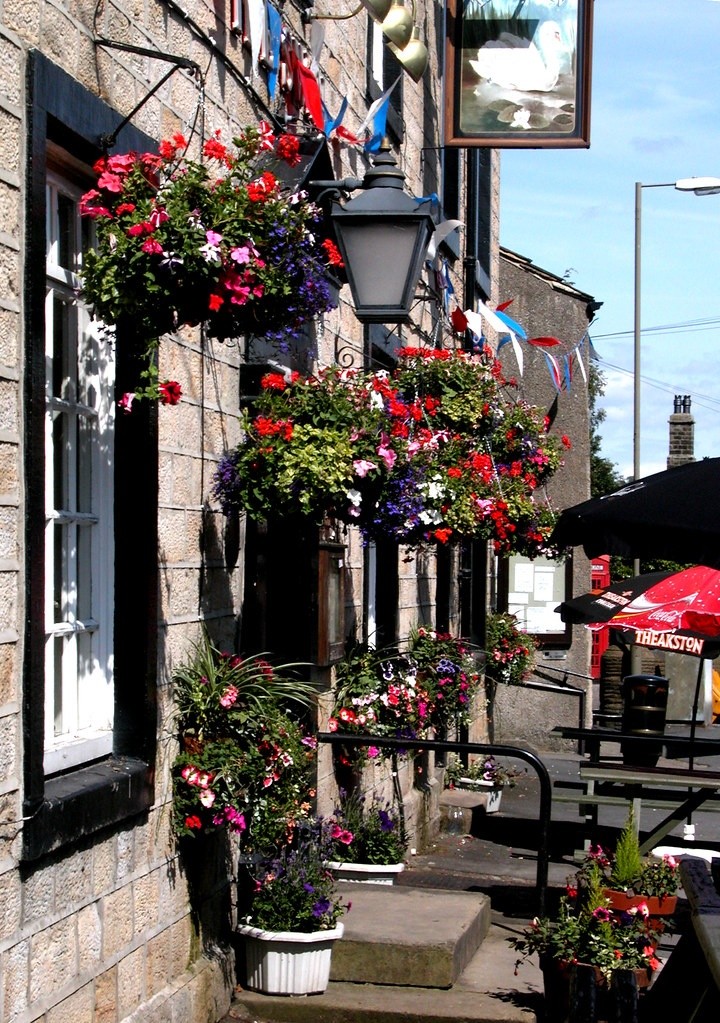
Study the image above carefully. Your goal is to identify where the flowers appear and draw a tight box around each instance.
[168,608,684,990]
[71,123,573,561]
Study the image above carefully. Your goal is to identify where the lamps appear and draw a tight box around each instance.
[386,38,429,83]
[303,132,436,325]
[382,0,415,51]
[304,0,393,24]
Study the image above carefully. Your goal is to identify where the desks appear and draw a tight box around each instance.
[550,724,720,828]
[578,760,720,789]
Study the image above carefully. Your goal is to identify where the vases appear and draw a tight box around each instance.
[455,777,510,812]
[322,860,405,885]
[234,922,345,997]
[538,955,651,1023]
[602,886,677,933]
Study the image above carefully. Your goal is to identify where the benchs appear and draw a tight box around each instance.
[553,779,720,799]
[551,792,720,813]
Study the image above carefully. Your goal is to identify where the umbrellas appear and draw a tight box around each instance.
[542,454,720,571]
[554,564,719,826]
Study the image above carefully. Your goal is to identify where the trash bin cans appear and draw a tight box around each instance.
[620,674,668,766]
